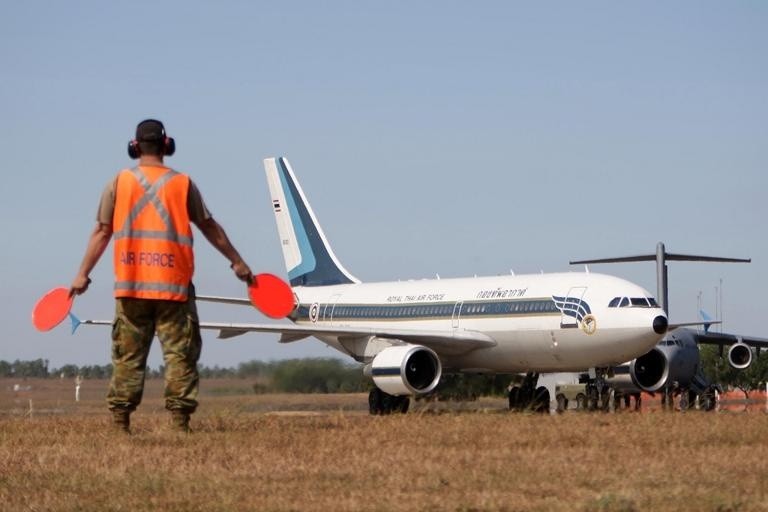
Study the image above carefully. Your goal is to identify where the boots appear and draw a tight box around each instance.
[113,408,129,438]
[171,410,192,432]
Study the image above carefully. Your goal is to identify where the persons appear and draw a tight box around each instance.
[66,117,260,434]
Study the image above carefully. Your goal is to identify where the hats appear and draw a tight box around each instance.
[137,120,166,142]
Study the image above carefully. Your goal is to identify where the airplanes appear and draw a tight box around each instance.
[69,157,668,413]
[570,242,768,411]
